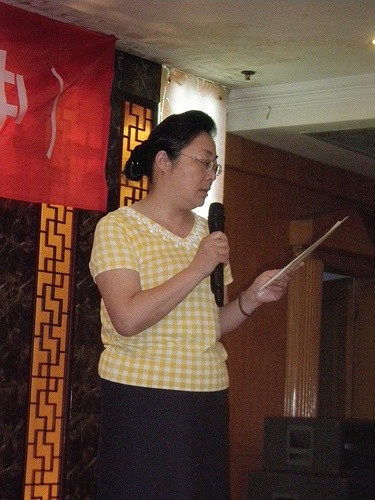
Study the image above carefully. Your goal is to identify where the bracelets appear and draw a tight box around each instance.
[238,291,256,317]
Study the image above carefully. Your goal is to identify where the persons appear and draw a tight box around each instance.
[88,110,306,500]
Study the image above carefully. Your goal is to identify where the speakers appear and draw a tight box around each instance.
[247,414,375,500]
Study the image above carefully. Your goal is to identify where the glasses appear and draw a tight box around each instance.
[178,150,222,176]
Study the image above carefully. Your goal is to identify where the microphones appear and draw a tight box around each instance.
[208,203,225,308]
